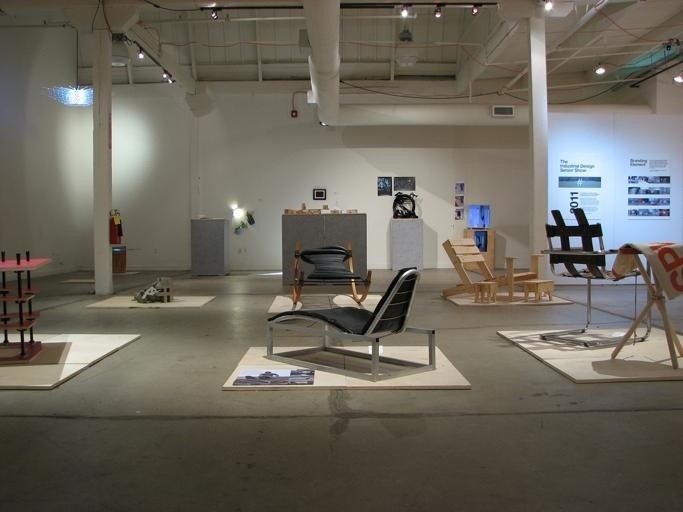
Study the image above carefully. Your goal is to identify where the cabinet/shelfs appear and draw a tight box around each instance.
[0,251,50,365]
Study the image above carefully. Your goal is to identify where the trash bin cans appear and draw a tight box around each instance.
[112,246,126,273]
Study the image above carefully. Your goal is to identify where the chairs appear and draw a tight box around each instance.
[263,266,436,383]
[291,238,375,305]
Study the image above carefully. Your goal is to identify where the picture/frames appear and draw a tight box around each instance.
[312,188,328,201]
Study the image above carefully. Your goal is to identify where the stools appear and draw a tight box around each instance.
[473,280,499,304]
[521,279,554,304]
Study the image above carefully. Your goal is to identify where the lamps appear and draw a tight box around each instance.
[470,4,482,22]
[594,61,609,78]
[432,5,444,21]
[399,5,411,18]
[134,44,148,60]
[45,86,95,109]
[208,7,222,20]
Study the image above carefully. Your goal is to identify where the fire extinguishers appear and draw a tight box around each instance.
[109,209,123,244]
